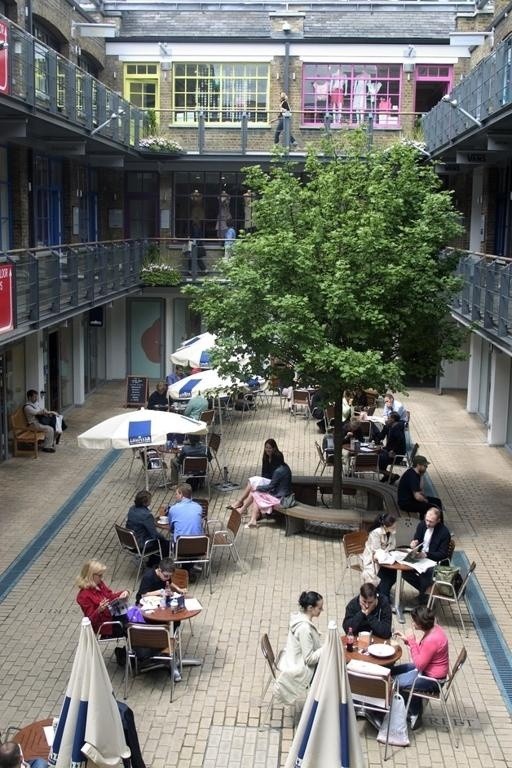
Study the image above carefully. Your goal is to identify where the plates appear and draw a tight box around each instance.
[157,520,169,524]
[140,596,158,606]
[361,447,373,452]
[369,644,395,657]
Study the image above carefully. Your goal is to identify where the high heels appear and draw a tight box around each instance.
[256,512,261,520]
[226,503,243,509]
[244,522,256,529]
[237,508,247,515]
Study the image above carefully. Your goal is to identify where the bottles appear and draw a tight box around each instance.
[160,588,165,606]
[165,581,171,596]
[347,627,354,652]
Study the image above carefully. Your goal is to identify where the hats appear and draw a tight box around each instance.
[413,456,430,465]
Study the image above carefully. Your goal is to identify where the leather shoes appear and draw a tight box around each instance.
[389,474,400,485]
[380,474,388,482]
[43,447,55,453]
[115,647,125,666]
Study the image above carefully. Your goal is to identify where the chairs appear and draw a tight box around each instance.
[314,441,346,476]
[336,532,370,596]
[199,410,214,427]
[259,633,285,733]
[206,508,246,578]
[178,456,211,500]
[352,454,380,481]
[139,446,167,492]
[401,647,467,748]
[191,498,209,533]
[342,668,396,761]
[254,379,269,407]
[124,623,175,703]
[348,421,370,439]
[424,561,476,638]
[169,535,212,595]
[111,523,163,591]
[324,407,337,435]
[289,390,311,422]
[386,443,420,484]
[171,568,194,636]
[206,434,223,479]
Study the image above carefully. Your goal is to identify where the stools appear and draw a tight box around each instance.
[96,621,133,677]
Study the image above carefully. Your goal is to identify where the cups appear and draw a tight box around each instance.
[159,516,168,521]
[357,630,372,657]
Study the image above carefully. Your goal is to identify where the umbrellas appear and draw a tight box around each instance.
[168,369,271,491]
[77,407,210,492]
[284,621,362,767]
[48,615,131,767]
[171,331,271,373]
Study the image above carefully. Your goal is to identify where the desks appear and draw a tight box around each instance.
[340,635,402,730]
[10,718,56,762]
[159,444,201,488]
[154,515,203,571]
[375,551,422,624]
[138,597,202,674]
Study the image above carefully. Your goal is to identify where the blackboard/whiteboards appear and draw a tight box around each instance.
[127,376,147,406]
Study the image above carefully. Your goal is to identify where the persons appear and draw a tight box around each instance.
[269,92,299,148]
[373,394,409,444]
[75,558,155,668]
[342,583,392,640]
[223,219,236,259]
[23,389,56,453]
[125,490,176,569]
[342,389,356,422]
[183,392,209,420]
[397,455,443,524]
[400,505,451,603]
[166,364,186,385]
[342,417,365,476]
[235,450,292,529]
[168,482,204,583]
[272,591,324,707]
[359,512,397,597]
[352,386,368,412]
[374,412,406,485]
[169,432,213,489]
[309,385,326,434]
[1,741,48,768]
[146,382,169,410]
[387,606,449,730]
[135,557,189,631]
[277,380,295,414]
[186,215,209,276]
[225,438,279,510]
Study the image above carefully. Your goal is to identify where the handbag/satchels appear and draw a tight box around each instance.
[55,415,63,432]
[279,493,296,509]
[377,694,409,747]
[23,403,51,425]
[432,558,461,596]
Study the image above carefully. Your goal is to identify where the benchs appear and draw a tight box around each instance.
[10,402,56,458]
[273,499,384,537]
[292,476,421,545]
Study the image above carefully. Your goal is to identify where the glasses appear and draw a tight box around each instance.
[94,574,103,577]
[162,572,173,578]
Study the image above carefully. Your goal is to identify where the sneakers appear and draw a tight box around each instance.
[410,712,420,729]
